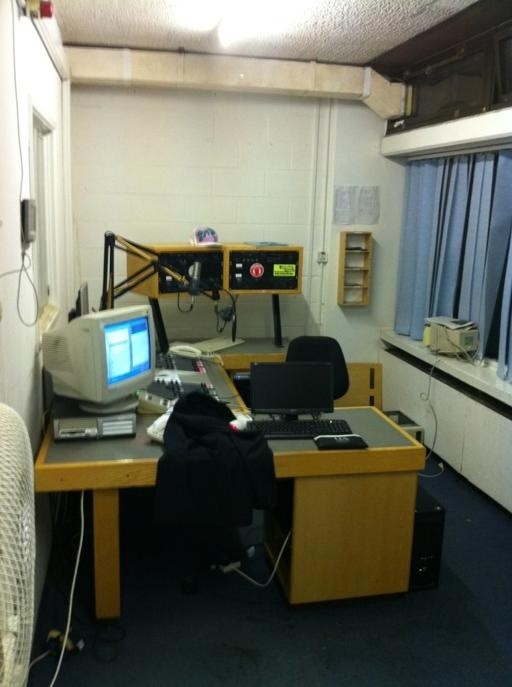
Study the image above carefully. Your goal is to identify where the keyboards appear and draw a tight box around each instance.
[246,418,353,440]
[193,335,246,353]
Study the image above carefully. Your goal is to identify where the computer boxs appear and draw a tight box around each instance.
[51,402,140,443]
[409,484,446,590]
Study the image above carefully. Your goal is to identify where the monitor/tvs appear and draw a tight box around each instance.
[249,360,334,420]
[66,281,91,322]
[41,305,157,415]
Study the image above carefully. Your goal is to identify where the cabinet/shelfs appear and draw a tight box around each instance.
[338,231,372,307]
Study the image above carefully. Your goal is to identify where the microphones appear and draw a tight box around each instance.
[185,260,203,296]
[218,303,234,323]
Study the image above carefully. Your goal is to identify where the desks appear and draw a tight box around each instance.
[34,337,426,618]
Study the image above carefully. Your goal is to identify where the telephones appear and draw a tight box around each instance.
[169,345,202,358]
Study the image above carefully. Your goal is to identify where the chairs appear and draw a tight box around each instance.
[285,337,349,401]
